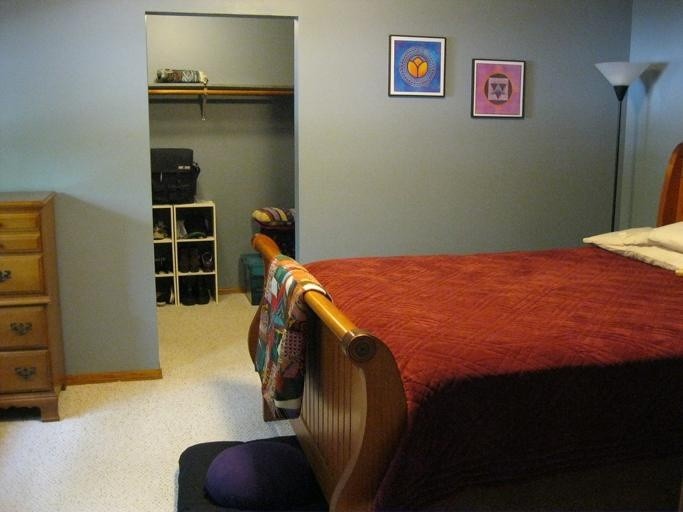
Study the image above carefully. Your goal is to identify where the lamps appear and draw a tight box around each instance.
[593,62,651,232]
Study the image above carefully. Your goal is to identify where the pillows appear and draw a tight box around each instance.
[177,436,330,512]
[205,440,312,511]
[649,220,683,253]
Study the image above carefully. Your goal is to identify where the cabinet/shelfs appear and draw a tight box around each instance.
[148,83,294,120]
[0,191,66,422]
[174,202,218,305]
[152,206,177,305]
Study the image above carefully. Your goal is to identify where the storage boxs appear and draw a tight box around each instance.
[248,264,266,306]
[239,253,265,289]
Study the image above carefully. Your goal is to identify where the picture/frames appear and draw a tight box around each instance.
[470,59,525,119]
[389,35,446,98]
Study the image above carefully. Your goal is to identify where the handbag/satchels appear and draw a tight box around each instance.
[151,148,198,205]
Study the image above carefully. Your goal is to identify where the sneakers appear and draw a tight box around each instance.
[157,294,165,306]
[201,253,214,271]
[165,284,175,304]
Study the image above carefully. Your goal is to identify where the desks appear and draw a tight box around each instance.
[255,221,294,257]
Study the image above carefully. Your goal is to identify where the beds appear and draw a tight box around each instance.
[248,143,683,512]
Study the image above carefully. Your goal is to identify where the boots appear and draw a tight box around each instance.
[191,248,201,272]
[197,276,209,304]
[182,277,196,305]
[162,246,170,270]
[154,246,162,274]
[179,247,190,272]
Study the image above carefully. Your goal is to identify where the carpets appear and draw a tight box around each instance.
[0,293,296,512]
